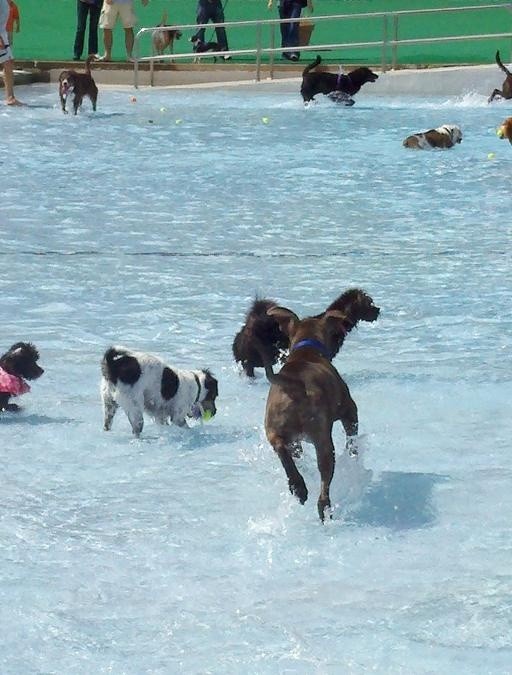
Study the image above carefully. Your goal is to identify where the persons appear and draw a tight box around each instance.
[192,0,233,64]
[94,0,151,62]
[6,0,24,70]
[267,0,314,62]
[1,0,27,108]
[73,0,105,61]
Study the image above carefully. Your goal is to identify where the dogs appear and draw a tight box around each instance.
[402,125,463,152]
[0,342,44,412]
[188,36,221,64]
[231,286,381,524]
[301,55,378,110]
[100,347,218,439]
[488,49,512,103]
[496,117,512,146]
[151,9,182,63]
[59,54,98,116]
